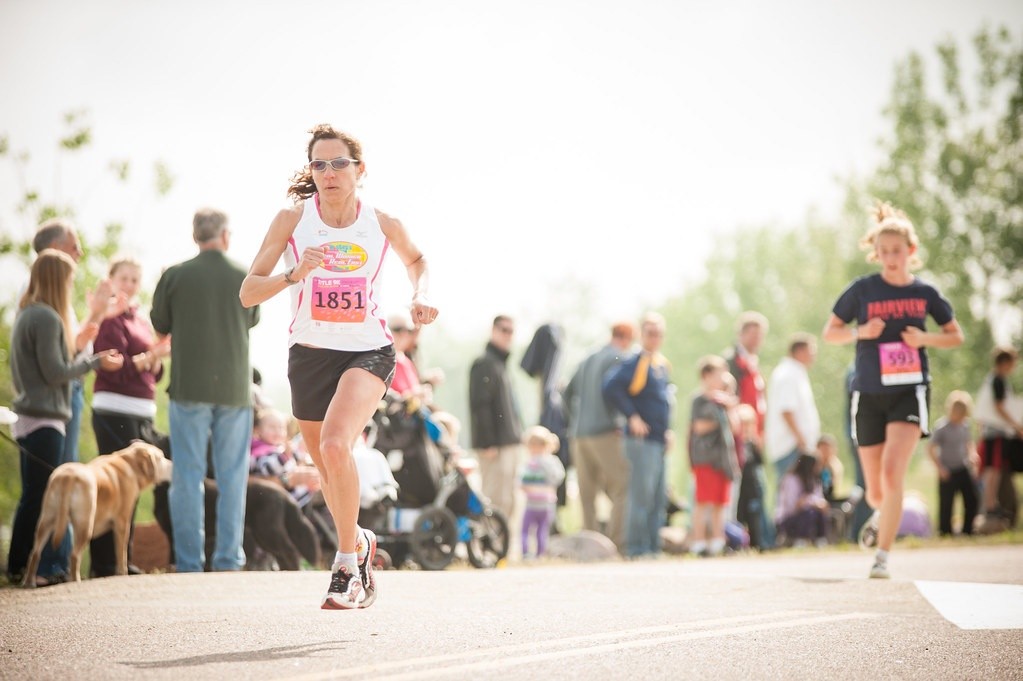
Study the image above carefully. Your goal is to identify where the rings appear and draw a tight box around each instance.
[431,317,435,321]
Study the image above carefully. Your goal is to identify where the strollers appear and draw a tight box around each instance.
[365,387,511,570]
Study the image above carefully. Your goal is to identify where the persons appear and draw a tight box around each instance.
[515,322,572,537]
[824,201,964,579]
[567,323,638,558]
[148,209,260,573]
[241,368,337,572]
[928,347,1023,537]
[8,209,170,590]
[603,313,674,559]
[523,424,565,559]
[468,316,522,554]
[658,313,872,554]
[351,306,477,571]
[237,126,440,611]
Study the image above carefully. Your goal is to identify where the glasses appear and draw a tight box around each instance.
[304,158,359,172]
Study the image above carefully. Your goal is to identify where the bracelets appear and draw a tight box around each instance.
[851,324,858,341]
[140,352,151,371]
[283,268,299,284]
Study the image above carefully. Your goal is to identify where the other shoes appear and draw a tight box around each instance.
[870,561,892,578]
[3,556,148,595]
[857,508,879,551]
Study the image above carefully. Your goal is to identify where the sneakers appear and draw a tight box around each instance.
[319,560,365,608]
[356,528,377,608]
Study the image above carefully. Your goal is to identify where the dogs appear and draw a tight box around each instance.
[20,439,172,589]
[145,421,318,573]
